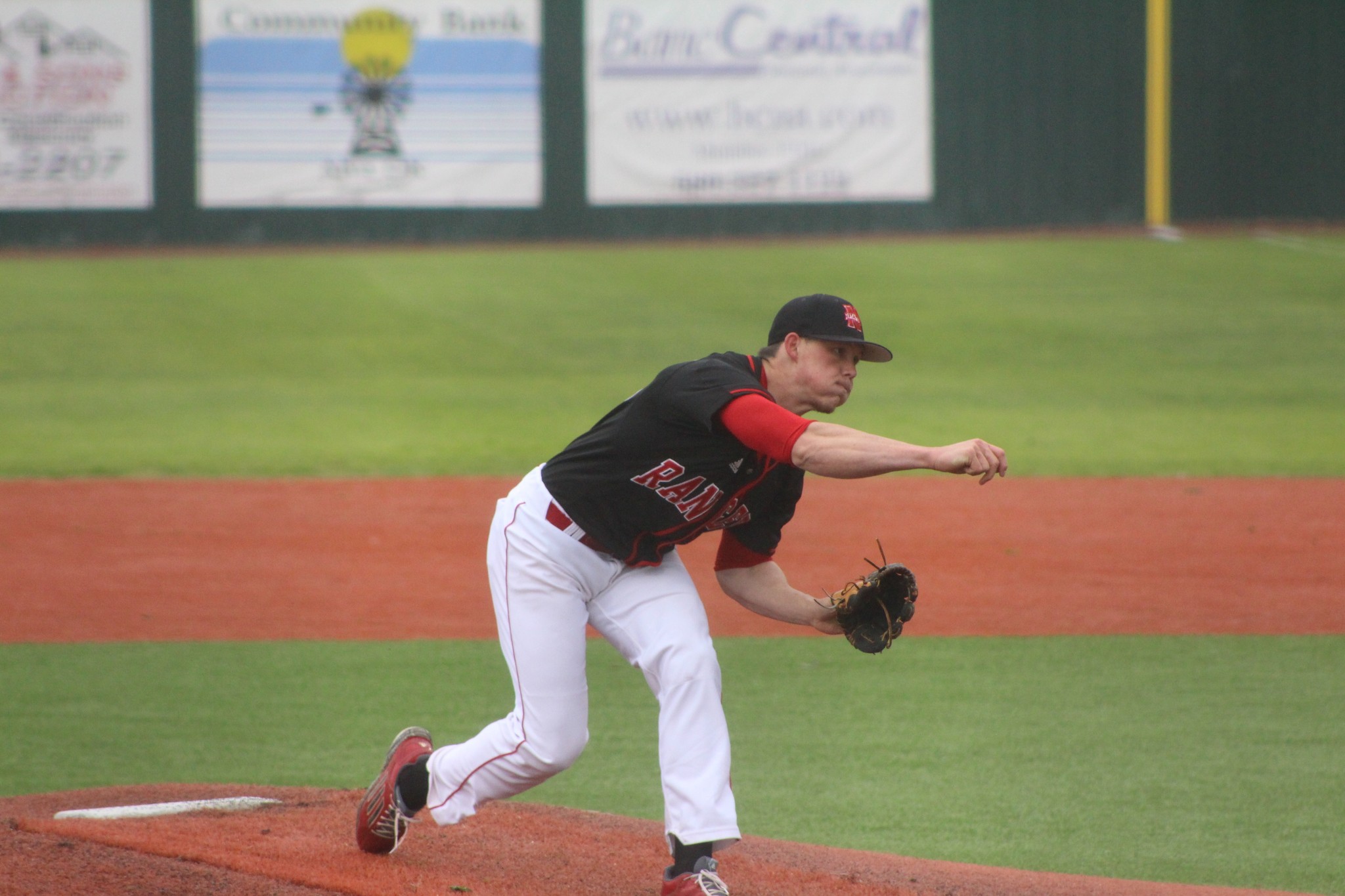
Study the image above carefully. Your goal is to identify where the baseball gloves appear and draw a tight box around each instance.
[831,561,918,654]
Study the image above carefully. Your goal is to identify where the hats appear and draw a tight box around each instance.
[767,292,893,363]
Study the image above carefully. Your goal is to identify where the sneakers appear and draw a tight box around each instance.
[357,726,436,855]
[661,856,730,896]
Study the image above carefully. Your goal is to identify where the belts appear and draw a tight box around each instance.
[546,502,608,555]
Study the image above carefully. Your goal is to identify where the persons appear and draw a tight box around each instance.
[356,293,1008,896]
